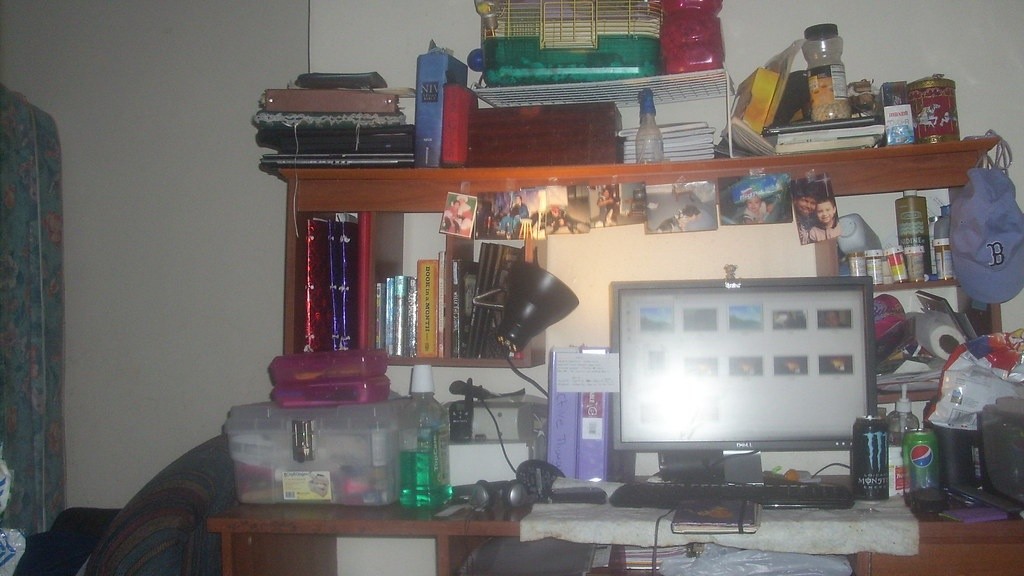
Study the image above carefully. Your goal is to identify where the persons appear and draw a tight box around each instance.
[742,188,775,225]
[597,188,618,227]
[476,196,529,239]
[795,187,842,245]
[441,195,474,236]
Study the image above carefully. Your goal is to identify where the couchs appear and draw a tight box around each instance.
[13,434,230,576]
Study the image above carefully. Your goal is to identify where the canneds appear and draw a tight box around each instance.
[903,428,941,491]
[850,414,890,503]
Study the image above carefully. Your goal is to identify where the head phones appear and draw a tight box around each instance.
[471,480,540,513]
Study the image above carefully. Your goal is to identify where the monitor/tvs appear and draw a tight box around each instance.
[610,274,877,485]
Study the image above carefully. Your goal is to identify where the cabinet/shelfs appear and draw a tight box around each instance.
[206,137,1024,576]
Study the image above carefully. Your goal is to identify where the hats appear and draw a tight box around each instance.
[949,167,1024,306]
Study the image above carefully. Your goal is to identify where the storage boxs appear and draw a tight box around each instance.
[222,350,410,507]
[474,0,662,88]
[468,101,623,166]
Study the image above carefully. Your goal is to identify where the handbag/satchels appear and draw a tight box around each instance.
[924,330,1024,431]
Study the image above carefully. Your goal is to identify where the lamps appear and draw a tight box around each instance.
[472,262,579,397]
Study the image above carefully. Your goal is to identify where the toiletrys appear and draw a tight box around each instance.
[399,363,452,508]
[885,382,919,495]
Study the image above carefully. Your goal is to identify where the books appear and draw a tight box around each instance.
[547,344,611,481]
[671,497,763,534]
[303,211,404,354]
[618,121,716,164]
[717,117,885,158]
[451,242,525,359]
[254,88,415,168]
[375,233,474,358]
[415,52,479,168]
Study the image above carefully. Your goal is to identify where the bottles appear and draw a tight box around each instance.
[847,190,955,286]
[802,23,852,123]
[397,365,453,508]
[636,88,663,165]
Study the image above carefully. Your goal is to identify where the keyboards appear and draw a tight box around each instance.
[609,480,855,512]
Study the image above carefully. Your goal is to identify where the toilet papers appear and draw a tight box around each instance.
[905,306,966,361]
